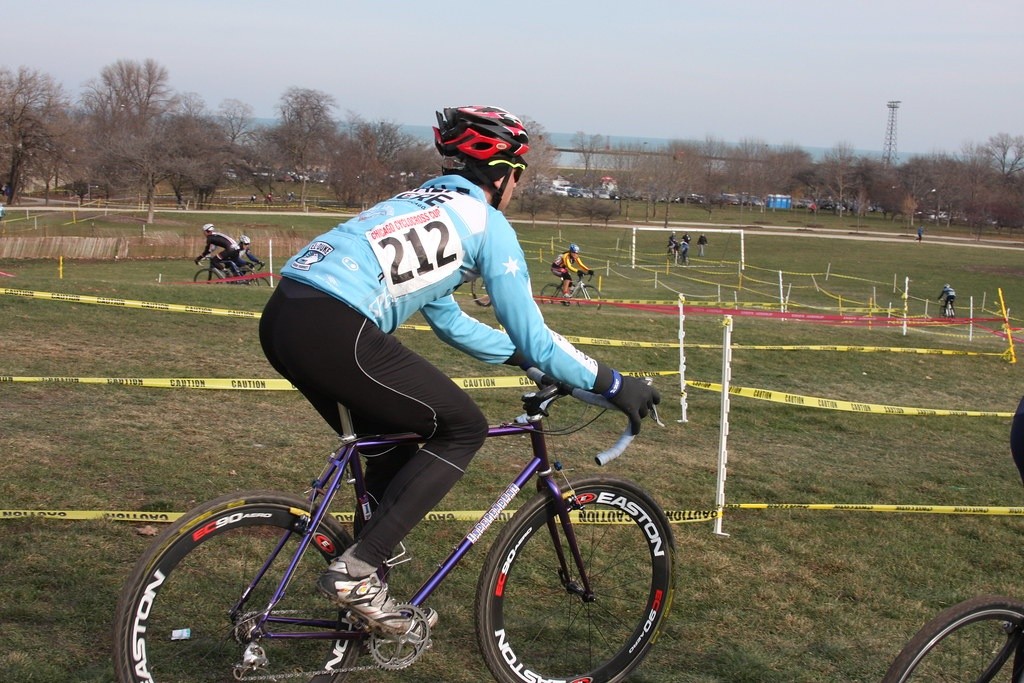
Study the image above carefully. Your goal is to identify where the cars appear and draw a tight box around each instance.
[598,191,610,199]
[722,193,739,205]
[554,187,568,196]
[993,217,1019,230]
[224,169,326,183]
[687,196,702,204]
[568,188,583,197]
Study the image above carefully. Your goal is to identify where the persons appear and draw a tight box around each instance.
[668,231,692,265]
[250,194,258,204]
[258,105,660,635]
[883,208,887,219]
[195,223,265,285]
[697,232,709,257]
[267,192,274,204]
[914,225,923,243]
[675,196,680,204]
[1010,394,1024,489]
[809,201,817,215]
[0,183,13,221]
[287,191,298,199]
[551,244,594,306]
[938,284,956,316]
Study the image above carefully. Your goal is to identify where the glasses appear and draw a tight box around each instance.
[488,159,525,182]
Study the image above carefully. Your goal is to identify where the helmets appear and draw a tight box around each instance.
[570,243,580,252]
[240,235,251,244]
[944,284,950,288]
[432,105,529,160]
[202,224,215,231]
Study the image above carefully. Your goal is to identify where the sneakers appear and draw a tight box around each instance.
[380,607,438,644]
[318,560,411,631]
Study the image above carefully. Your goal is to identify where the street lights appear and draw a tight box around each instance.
[920,188,936,225]
[88,184,99,208]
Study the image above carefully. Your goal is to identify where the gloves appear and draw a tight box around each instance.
[259,261,265,266]
[502,348,532,371]
[194,255,203,265]
[588,270,594,275]
[590,361,660,434]
[577,270,583,276]
[246,262,255,269]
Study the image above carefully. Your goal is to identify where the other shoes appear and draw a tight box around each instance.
[563,294,570,305]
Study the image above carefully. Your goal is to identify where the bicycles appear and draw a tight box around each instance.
[194,246,271,287]
[666,248,690,267]
[881,594,1024,683]
[471,276,493,307]
[938,297,956,318]
[540,272,602,310]
[113,368,675,683]
